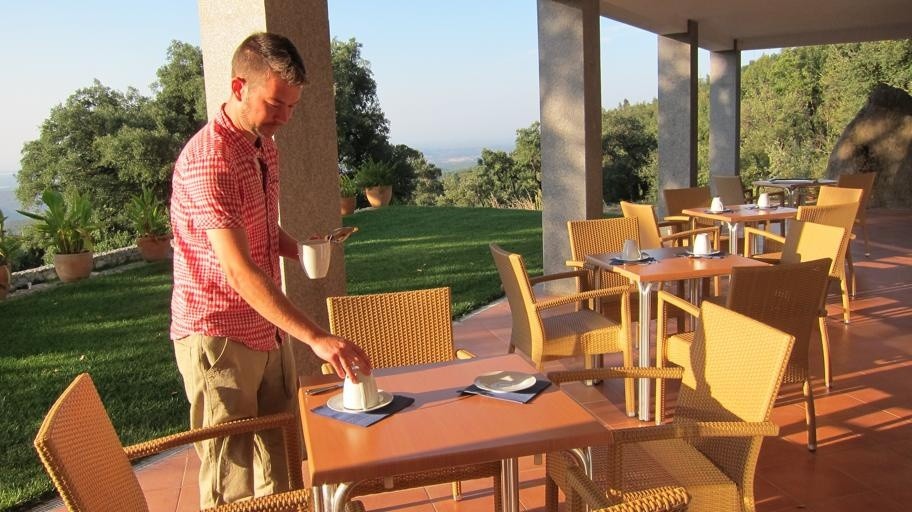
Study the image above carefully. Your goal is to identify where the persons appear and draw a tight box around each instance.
[170,31,374,509]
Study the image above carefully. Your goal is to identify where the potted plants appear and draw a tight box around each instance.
[0,210,12,300]
[15,183,97,283]
[339,171,357,216]
[120,181,173,262]
[353,152,398,207]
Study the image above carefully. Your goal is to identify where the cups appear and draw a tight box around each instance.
[759,194,769,208]
[693,234,711,254]
[624,240,641,258]
[342,367,383,410]
[710,197,724,211]
[297,239,332,279]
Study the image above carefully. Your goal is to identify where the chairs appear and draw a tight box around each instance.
[544,299,797,512]
[320,287,479,500]
[33,371,317,512]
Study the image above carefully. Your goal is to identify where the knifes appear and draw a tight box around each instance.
[309,382,344,395]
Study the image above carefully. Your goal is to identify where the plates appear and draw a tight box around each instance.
[327,387,393,414]
[704,209,730,213]
[474,370,537,394]
[610,252,650,261]
[687,250,720,257]
